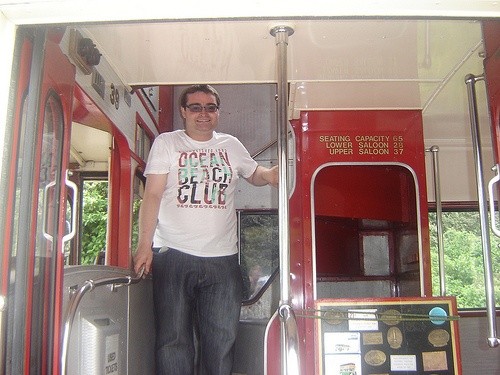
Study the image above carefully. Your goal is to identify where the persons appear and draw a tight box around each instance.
[133,84,281,375]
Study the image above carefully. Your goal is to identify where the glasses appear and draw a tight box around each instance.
[185,104,219,112]
